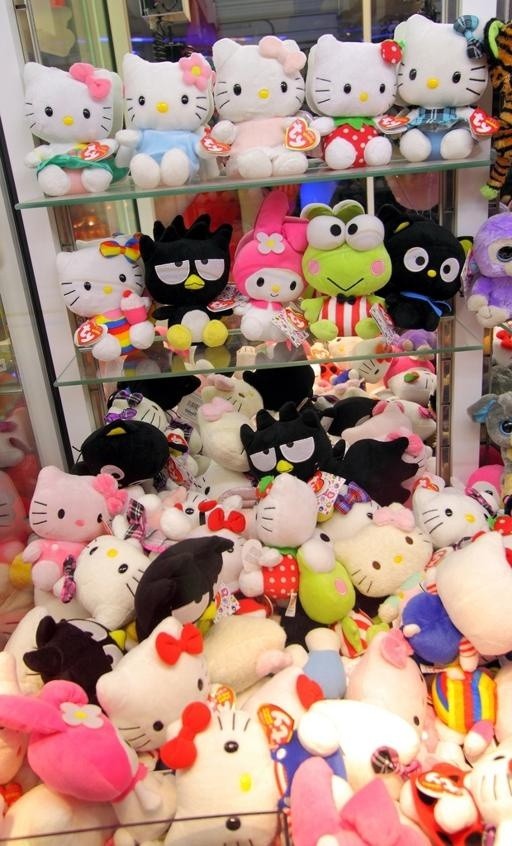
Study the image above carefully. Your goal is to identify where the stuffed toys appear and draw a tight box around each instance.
[0,14,512,839]
[0,14,512,846]
